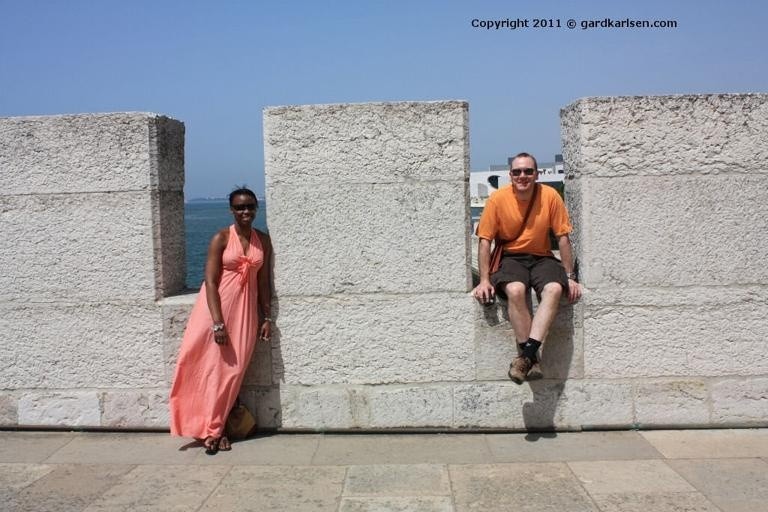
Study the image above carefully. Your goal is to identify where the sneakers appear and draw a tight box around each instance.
[527,358,543,379]
[508,357,532,385]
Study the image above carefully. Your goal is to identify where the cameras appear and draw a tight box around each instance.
[482,289,497,307]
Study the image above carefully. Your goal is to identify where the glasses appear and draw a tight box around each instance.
[232,203,255,212]
[511,168,534,177]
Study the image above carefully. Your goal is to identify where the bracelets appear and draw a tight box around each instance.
[566,272,576,280]
[263,318,272,322]
[210,322,224,332]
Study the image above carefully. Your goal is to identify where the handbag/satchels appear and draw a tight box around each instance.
[225,397,257,440]
[489,240,505,273]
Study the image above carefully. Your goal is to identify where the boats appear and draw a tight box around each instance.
[469,155,568,281]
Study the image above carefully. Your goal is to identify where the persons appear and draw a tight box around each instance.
[469,152,581,384]
[167,183,273,454]
